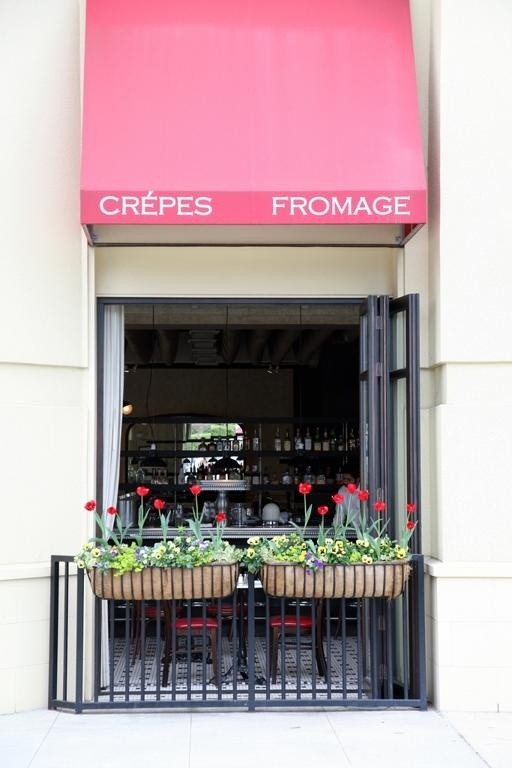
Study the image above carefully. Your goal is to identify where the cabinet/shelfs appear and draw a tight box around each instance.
[121,413,360,528]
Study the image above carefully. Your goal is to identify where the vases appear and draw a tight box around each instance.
[259,560,411,598]
[88,564,238,601]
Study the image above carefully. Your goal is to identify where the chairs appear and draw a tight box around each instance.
[132,600,171,665]
[206,602,239,641]
[162,601,219,687]
[269,598,326,684]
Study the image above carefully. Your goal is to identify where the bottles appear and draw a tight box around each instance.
[128,462,345,487]
[232,503,247,527]
[198,423,359,453]
[238,572,248,586]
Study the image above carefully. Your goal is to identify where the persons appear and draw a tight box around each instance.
[330,461,361,529]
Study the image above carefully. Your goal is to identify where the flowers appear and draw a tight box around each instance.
[246,482,420,577]
[72,483,245,579]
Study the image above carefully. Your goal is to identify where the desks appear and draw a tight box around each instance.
[208,573,267,686]
